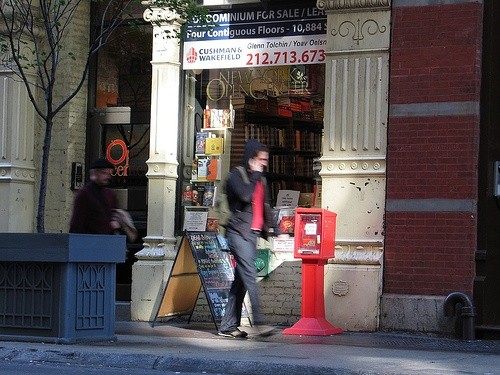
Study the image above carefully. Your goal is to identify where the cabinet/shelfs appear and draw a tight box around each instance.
[235,108,324,209]
[189,108,235,207]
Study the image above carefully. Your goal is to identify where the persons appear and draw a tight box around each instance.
[68,158,124,235]
[218,139,275,338]
[192,189,200,205]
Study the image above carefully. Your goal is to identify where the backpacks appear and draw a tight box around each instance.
[216,166,249,239]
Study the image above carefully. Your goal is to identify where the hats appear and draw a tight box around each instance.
[87,158,114,170]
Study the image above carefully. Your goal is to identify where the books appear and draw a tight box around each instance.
[182,109,322,232]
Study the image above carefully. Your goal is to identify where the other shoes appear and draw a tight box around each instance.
[255,324,275,335]
[217,328,248,338]
